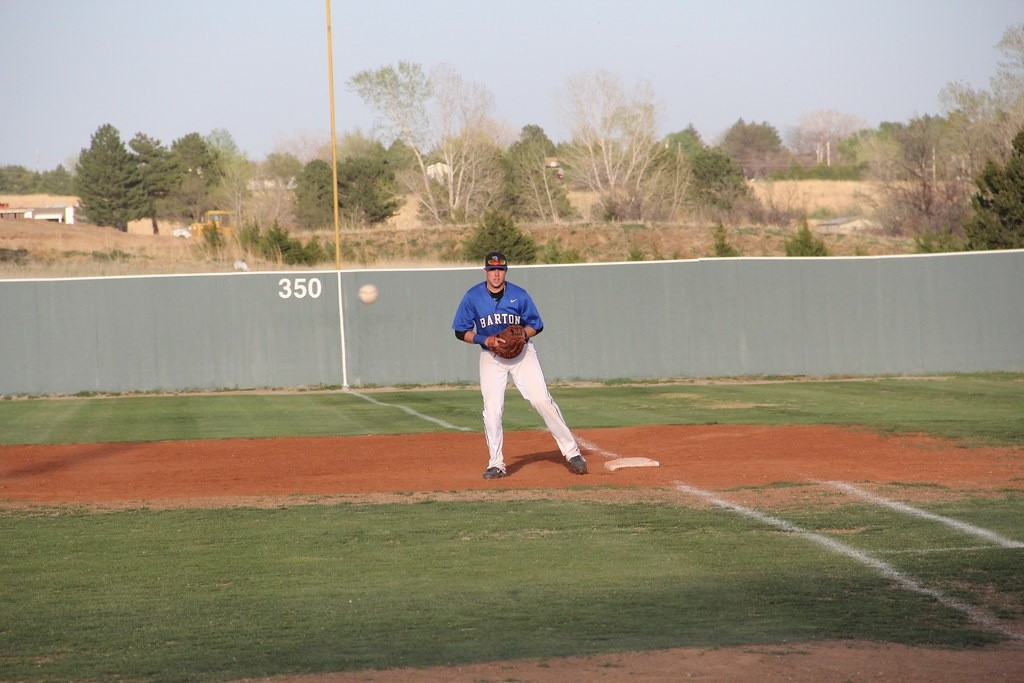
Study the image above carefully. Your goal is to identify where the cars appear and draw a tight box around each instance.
[173,228,191,238]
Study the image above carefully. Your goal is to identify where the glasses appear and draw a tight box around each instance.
[484,259,507,266]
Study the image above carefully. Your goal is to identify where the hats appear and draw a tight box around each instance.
[484,252,507,270]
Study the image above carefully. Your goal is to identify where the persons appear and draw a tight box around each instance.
[452,251,587,479]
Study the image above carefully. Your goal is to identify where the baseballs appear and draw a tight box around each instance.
[357,284,378,303]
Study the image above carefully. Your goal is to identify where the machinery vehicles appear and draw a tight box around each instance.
[190,210,236,239]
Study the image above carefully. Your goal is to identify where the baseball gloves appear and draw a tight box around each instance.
[489,325,525,360]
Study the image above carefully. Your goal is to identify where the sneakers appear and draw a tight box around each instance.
[483,466,504,479]
[568,454,587,475]
